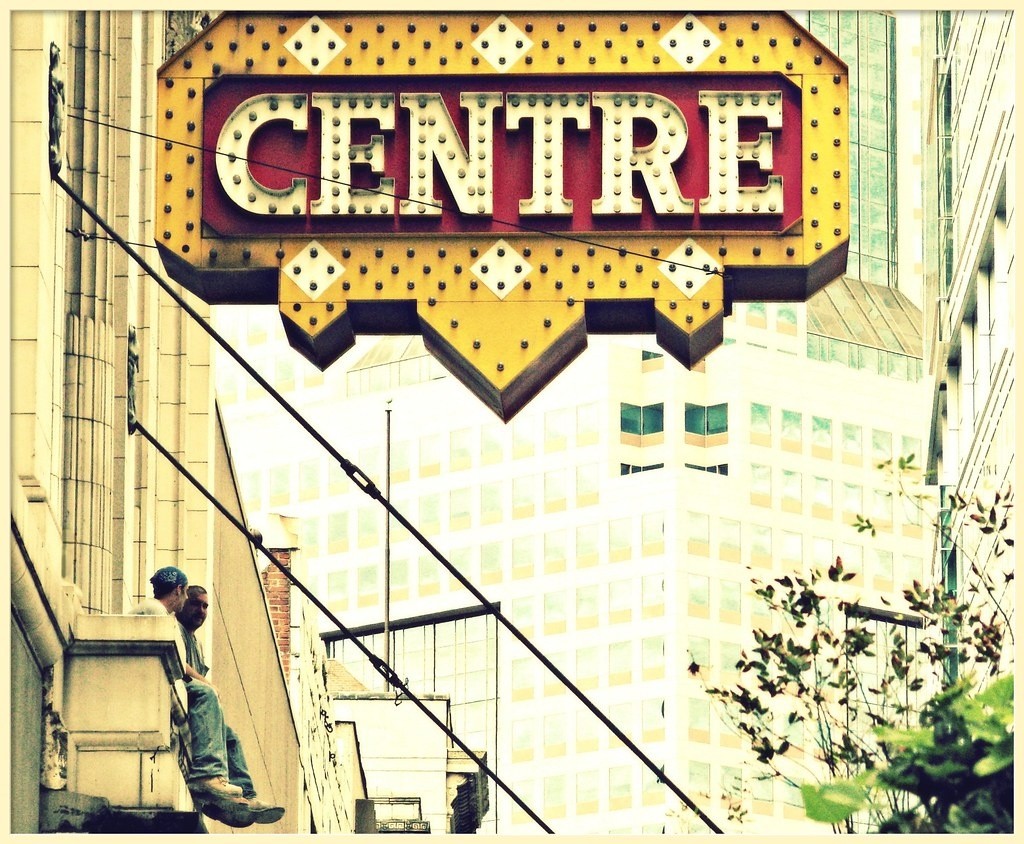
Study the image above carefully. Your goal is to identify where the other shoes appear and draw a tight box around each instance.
[193,793,249,811]
[187,775,243,797]
[245,797,285,824]
[197,805,254,828]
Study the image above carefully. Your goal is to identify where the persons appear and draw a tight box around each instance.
[120,566,285,827]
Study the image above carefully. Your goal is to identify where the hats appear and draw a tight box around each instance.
[150,567,188,598]
